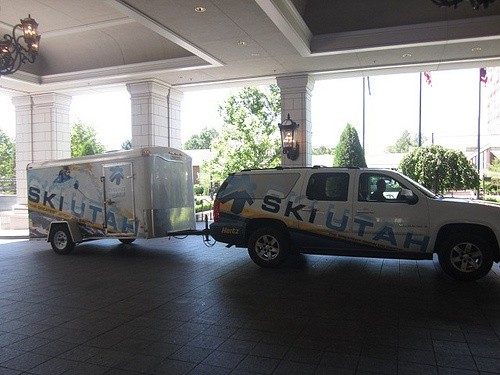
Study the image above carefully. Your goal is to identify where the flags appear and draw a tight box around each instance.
[424,71,432,87]
[480,68,488,84]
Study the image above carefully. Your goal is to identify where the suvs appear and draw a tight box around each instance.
[209,165,500,281]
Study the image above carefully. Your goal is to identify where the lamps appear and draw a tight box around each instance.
[0,13,41,74]
[278,113,300,161]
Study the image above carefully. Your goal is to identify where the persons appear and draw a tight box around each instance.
[370,179,388,201]
